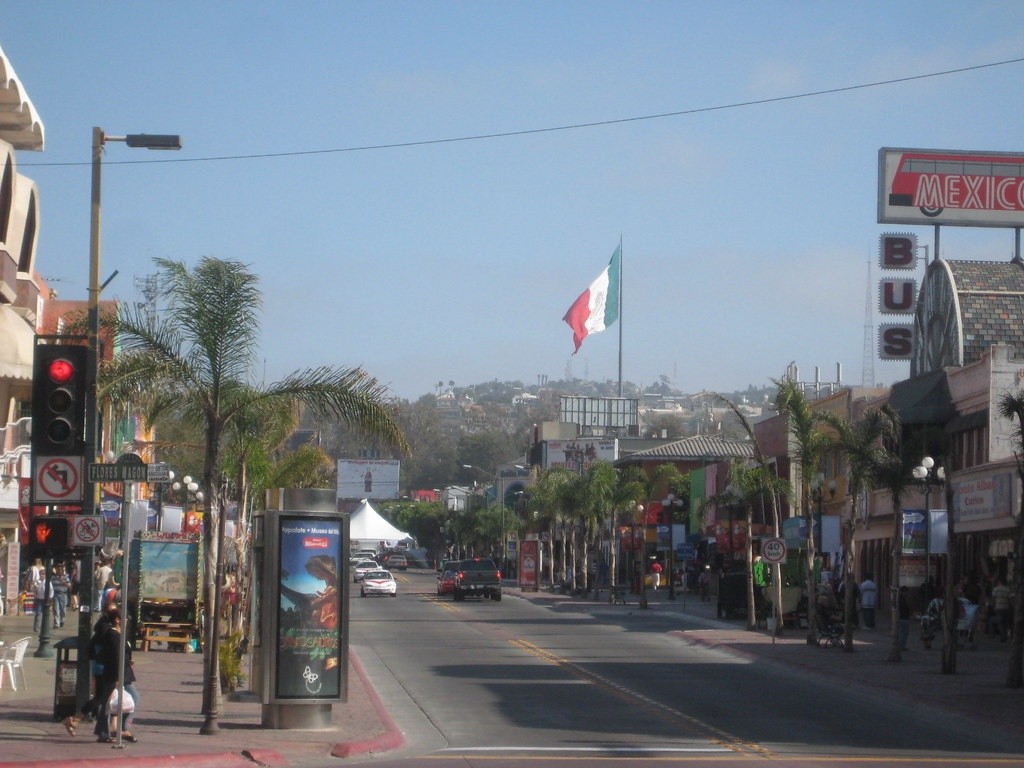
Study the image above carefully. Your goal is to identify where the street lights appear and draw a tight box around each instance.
[74,127,182,715]
[913,457,947,614]
[178,475,203,533]
[433,488,458,509]
[462,464,506,581]
[662,494,683,585]
[720,486,744,564]
[810,472,836,571]
[146,469,181,532]
[623,500,644,594]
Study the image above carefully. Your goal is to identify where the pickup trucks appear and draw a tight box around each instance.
[454,558,502,602]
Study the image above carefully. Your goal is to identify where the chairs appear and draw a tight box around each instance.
[0,636,33,691]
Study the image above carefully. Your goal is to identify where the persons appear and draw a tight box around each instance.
[543,551,610,597]
[25,554,80,633]
[94,550,137,650]
[898,585,913,653]
[442,541,518,580]
[141,602,197,653]
[785,565,880,631]
[955,550,1019,644]
[63,600,139,744]
[632,550,719,607]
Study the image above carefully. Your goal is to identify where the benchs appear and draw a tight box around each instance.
[609,585,627,605]
[140,627,190,634]
[813,613,844,649]
[144,636,189,643]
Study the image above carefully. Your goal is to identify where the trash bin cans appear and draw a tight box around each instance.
[52,637,95,722]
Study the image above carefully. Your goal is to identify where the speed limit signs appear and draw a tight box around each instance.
[761,538,787,563]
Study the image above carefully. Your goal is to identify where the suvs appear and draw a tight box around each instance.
[385,554,408,570]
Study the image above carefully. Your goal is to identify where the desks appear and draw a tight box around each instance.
[139,623,192,654]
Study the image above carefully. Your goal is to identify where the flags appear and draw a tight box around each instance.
[563,243,620,354]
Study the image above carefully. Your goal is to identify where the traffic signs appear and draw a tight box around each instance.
[87,461,170,482]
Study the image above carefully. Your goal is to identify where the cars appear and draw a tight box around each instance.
[361,570,397,597]
[349,548,378,564]
[353,559,383,582]
[437,562,459,596]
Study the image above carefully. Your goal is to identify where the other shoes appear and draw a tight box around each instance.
[61,621,64,627]
[97,738,116,742]
[62,716,78,737]
[53,626,59,629]
[122,734,138,743]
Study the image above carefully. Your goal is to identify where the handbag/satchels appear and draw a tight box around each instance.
[105,686,135,715]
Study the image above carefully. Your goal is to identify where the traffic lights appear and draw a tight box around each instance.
[31,344,88,453]
[29,517,69,559]
